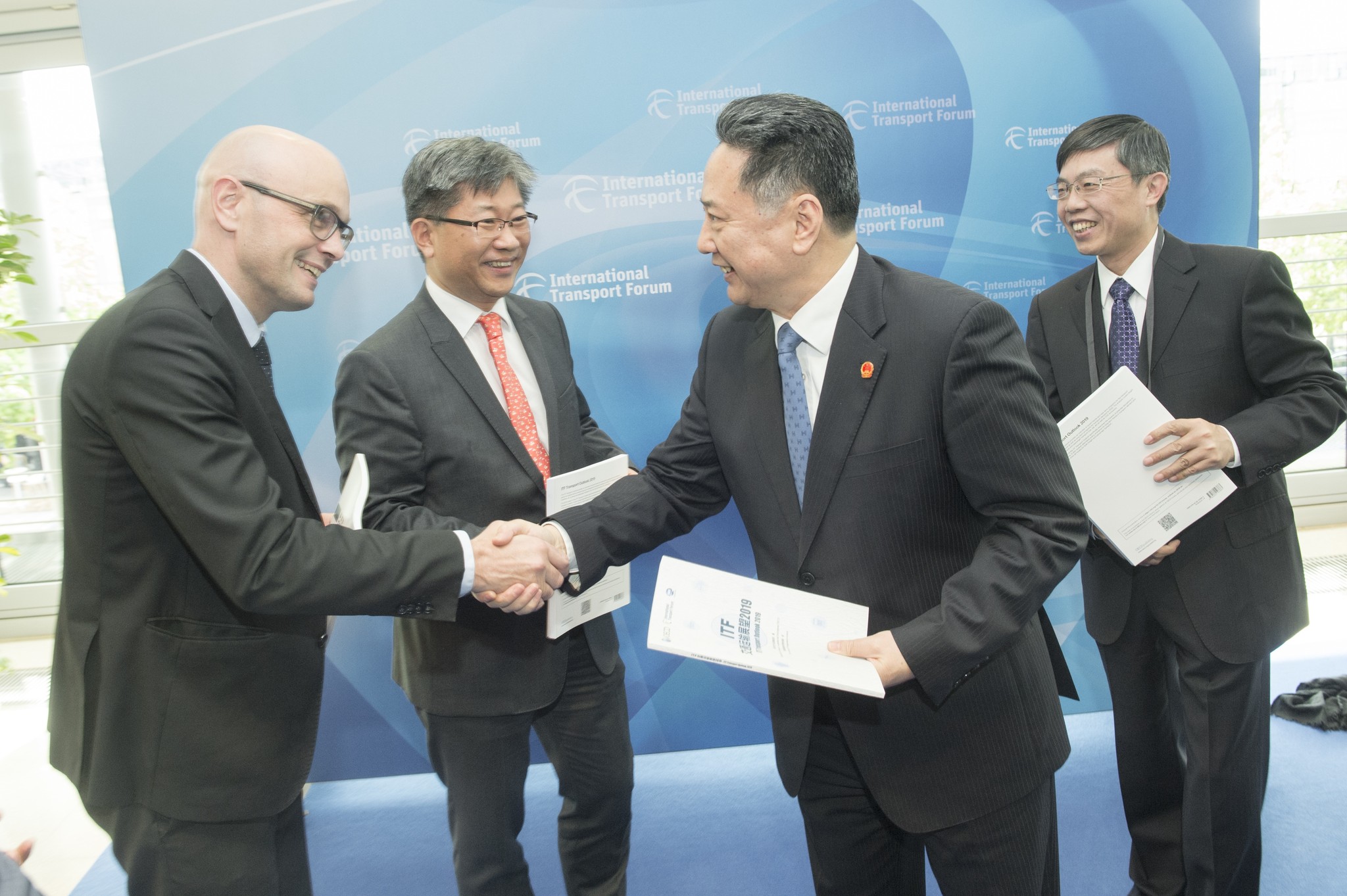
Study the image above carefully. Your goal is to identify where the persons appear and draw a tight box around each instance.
[473,91,1089,896]
[331,136,646,896]
[48,122,572,896]
[1025,116,1347,893]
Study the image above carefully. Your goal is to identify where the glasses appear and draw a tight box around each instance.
[1045,173,1148,201]
[426,211,538,239]
[239,180,355,252]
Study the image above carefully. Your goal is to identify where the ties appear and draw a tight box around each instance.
[254,337,276,397]
[476,312,552,492]
[1108,278,1140,376]
[776,321,812,516]
